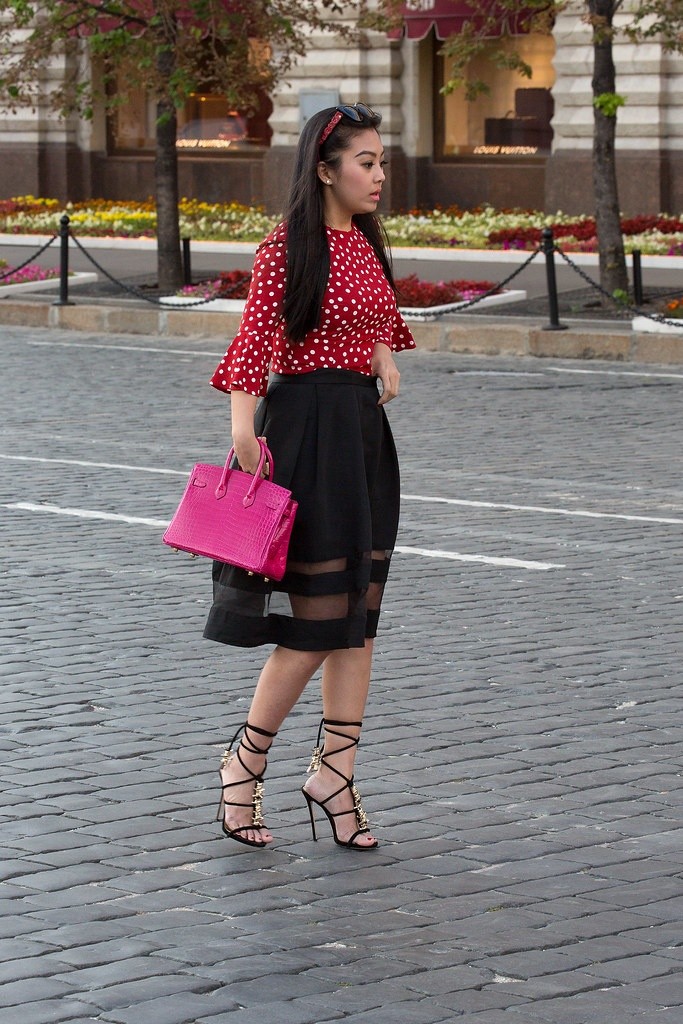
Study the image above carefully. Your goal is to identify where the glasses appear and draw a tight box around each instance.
[317,101,375,147]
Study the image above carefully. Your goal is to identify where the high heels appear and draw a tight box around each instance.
[217,721,268,848]
[301,719,379,851]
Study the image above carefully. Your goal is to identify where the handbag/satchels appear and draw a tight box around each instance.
[162,439,298,582]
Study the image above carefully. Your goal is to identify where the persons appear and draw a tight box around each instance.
[212,107,414,852]
